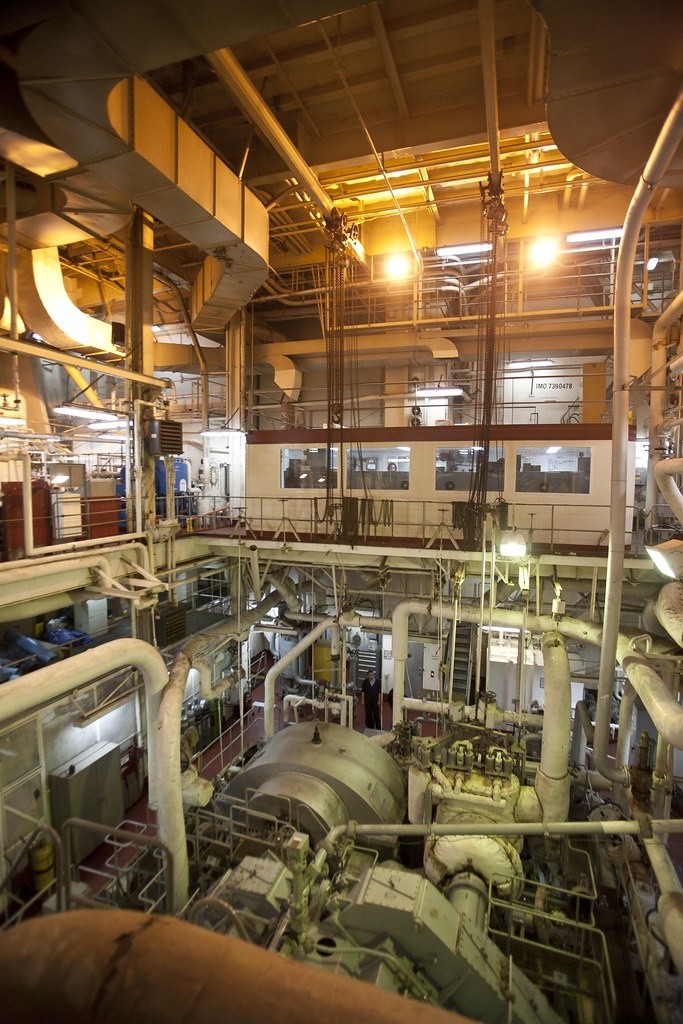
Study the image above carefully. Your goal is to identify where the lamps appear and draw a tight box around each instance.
[499,504,526,558]
[50,473,70,483]
[644,539,683,580]
[74,693,134,728]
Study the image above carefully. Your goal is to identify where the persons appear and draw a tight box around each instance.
[362,670,381,730]
[388,463,397,472]
